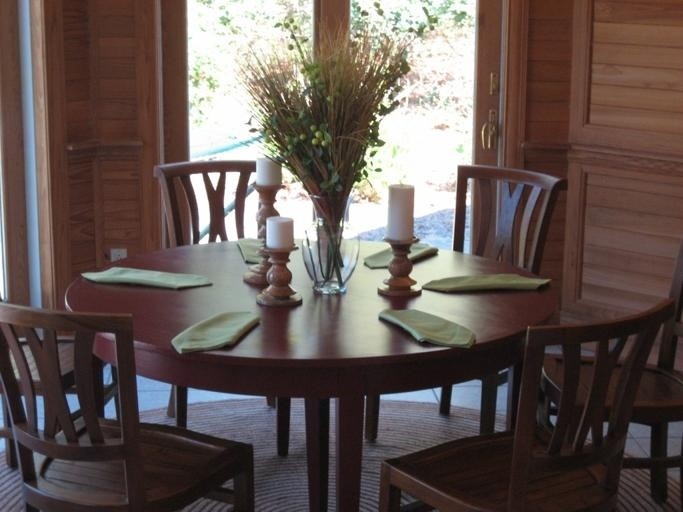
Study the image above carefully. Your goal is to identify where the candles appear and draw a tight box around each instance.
[265,217,295,248]
[255,160,283,186]
[387,184,415,240]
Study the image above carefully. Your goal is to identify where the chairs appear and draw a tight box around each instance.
[380,298,675,511]
[154,162,281,415]
[0,337,121,469]
[541,252,680,504]
[439,165,564,435]
[2,301,254,510]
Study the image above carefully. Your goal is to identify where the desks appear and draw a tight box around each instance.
[65,238,561,510]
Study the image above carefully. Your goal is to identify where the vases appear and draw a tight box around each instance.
[298,187,361,295]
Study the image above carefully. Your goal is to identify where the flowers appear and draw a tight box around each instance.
[236,1,438,296]
